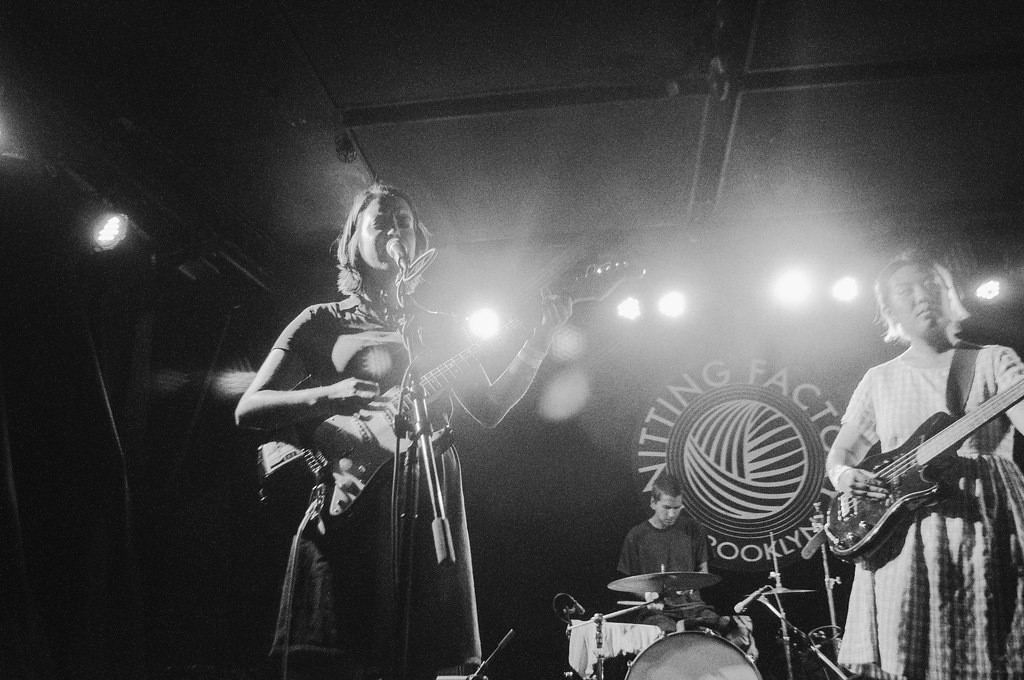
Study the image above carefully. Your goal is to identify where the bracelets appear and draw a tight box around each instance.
[517,339,548,370]
[831,465,850,492]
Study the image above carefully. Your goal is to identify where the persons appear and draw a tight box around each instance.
[823,254,1024,680]
[617,475,721,636]
[234,183,573,680]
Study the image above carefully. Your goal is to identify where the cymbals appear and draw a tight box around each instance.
[605,571,724,596]
[744,587,816,596]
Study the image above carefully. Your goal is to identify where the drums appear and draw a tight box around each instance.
[623,628,761,680]
[677,614,760,667]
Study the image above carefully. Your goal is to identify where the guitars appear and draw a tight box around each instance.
[820,377,1024,567]
[307,244,647,529]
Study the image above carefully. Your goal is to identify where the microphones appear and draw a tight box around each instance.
[734,585,769,613]
[386,238,410,269]
[567,594,585,615]
